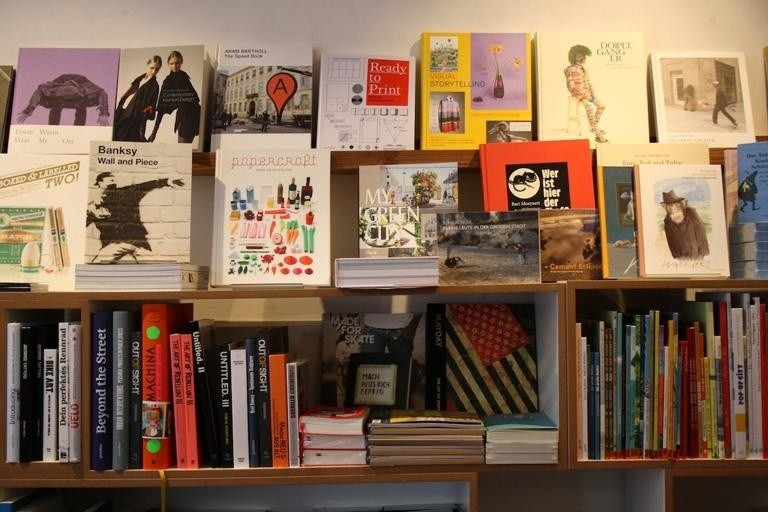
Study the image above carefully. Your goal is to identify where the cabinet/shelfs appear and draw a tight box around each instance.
[1,280,768,511]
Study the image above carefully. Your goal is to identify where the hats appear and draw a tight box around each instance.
[659,191,684,203]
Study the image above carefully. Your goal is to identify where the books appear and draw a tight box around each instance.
[575,289,767,462]
[88,297,560,472]
[3,309,84,467]
[0,30,768,292]
